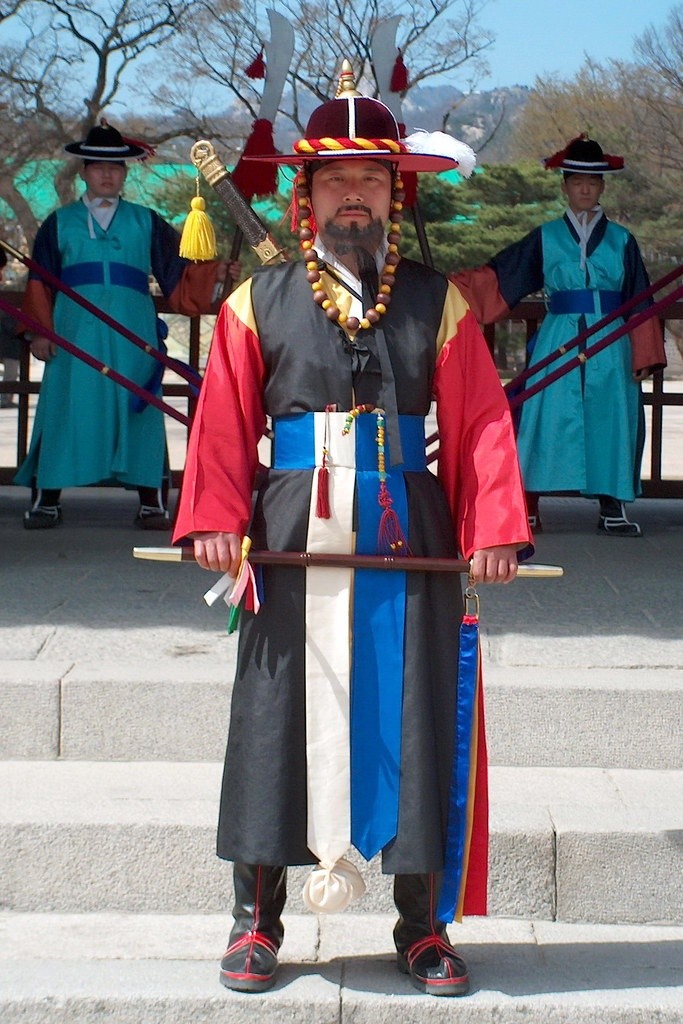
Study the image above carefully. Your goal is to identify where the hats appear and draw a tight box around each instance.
[62,118,155,163]
[242,59,476,178]
[542,132,627,174]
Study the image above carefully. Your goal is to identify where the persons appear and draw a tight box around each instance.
[171,60,538,995]
[15,117,242,528]
[448,130,668,535]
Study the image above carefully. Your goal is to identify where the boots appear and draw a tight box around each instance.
[136,475,171,531]
[219,860,288,992]
[393,871,471,996]
[597,493,641,537]
[526,493,541,534]
[23,472,63,529]
